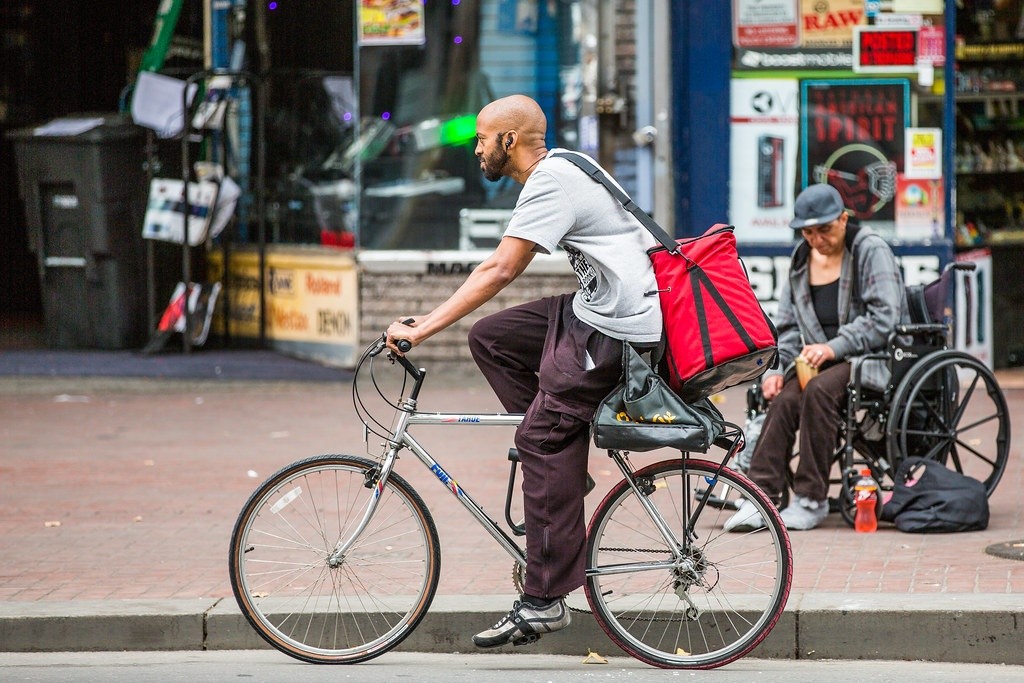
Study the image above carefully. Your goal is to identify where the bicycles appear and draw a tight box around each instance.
[230,318,792,670]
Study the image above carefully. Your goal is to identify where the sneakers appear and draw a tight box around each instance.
[510,470,597,537]
[471,596,572,648]
[724,499,764,532]
[779,494,830,530]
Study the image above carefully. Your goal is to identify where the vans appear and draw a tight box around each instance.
[257,65,509,234]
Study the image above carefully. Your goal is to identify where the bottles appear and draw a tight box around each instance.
[854,469,878,532]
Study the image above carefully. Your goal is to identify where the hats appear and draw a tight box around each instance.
[787,184,844,228]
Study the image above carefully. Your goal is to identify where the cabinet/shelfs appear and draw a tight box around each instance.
[913,43,1024,249]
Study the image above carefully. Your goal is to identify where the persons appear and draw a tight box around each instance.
[724,184,912,531]
[385,93,663,649]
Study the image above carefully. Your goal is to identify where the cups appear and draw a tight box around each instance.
[796,356,819,391]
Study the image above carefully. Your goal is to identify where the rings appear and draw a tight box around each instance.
[816,348,822,355]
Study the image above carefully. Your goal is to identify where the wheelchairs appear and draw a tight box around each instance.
[693,258,1010,528]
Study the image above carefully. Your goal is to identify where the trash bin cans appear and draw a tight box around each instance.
[2,112,190,350]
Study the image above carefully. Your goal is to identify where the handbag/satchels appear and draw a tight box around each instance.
[646,223,779,404]
[879,456,991,535]
